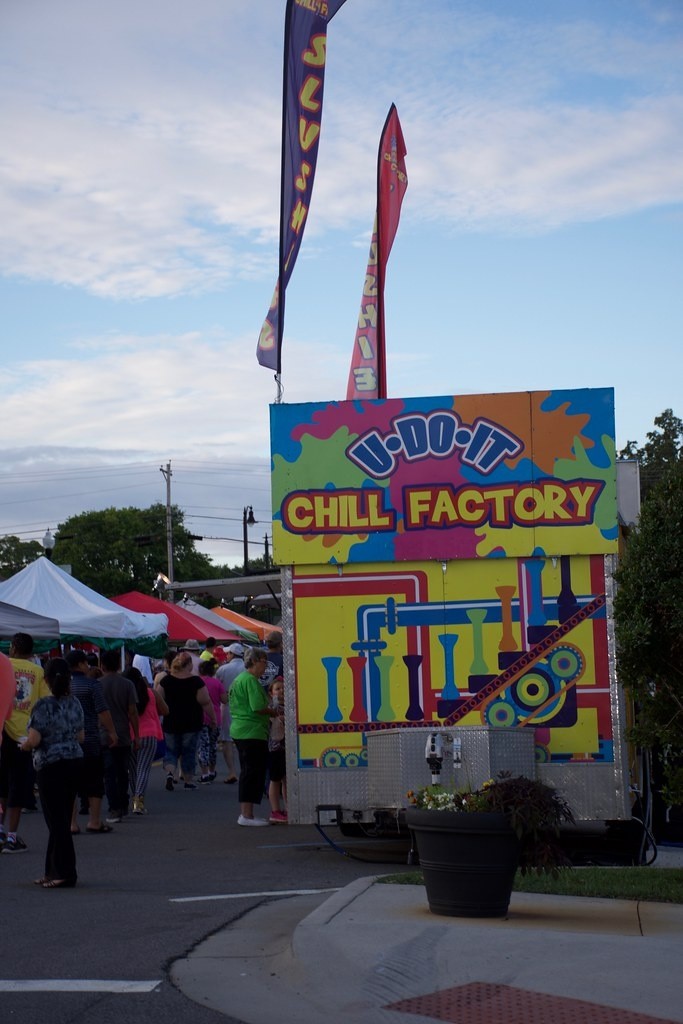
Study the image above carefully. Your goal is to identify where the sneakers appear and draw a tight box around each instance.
[197,776,210,784]
[210,771,218,782]
[2,837,28,853]
[0,833,7,845]
[269,811,287,824]
[238,815,269,826]
[224,773,238,783]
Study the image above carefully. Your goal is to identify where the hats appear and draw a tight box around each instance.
[66,650,93,663]
[223,642,244,656]
[179,639,202,650]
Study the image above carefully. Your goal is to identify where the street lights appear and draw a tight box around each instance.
[242,505,256,569]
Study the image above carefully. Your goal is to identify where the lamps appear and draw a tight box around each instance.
[151,572,171,594]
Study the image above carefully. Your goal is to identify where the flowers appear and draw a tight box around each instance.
[407,766,579,880]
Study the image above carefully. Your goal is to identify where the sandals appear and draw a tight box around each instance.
[34,875,50,883]
[44,879,70,888]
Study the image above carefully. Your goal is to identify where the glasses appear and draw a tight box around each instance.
[260,660,267,666]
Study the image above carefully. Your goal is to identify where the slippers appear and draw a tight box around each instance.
[86,820,114,834]
[71,824,80,834]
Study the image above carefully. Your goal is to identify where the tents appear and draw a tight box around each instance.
[0,556,282,673]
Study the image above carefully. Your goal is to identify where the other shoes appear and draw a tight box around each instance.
[21,805,38,813]
[79,808,89,815]
[184,783,199,790]
[166,774,174,791]
[106,810,122,823]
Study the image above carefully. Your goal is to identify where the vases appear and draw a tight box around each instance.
[407,806,517,918]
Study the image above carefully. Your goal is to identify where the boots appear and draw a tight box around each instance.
[133,794,148,815]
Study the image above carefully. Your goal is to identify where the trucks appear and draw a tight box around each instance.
[268,386,655,870]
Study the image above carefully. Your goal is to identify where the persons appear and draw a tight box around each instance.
[0,631,286,888]
